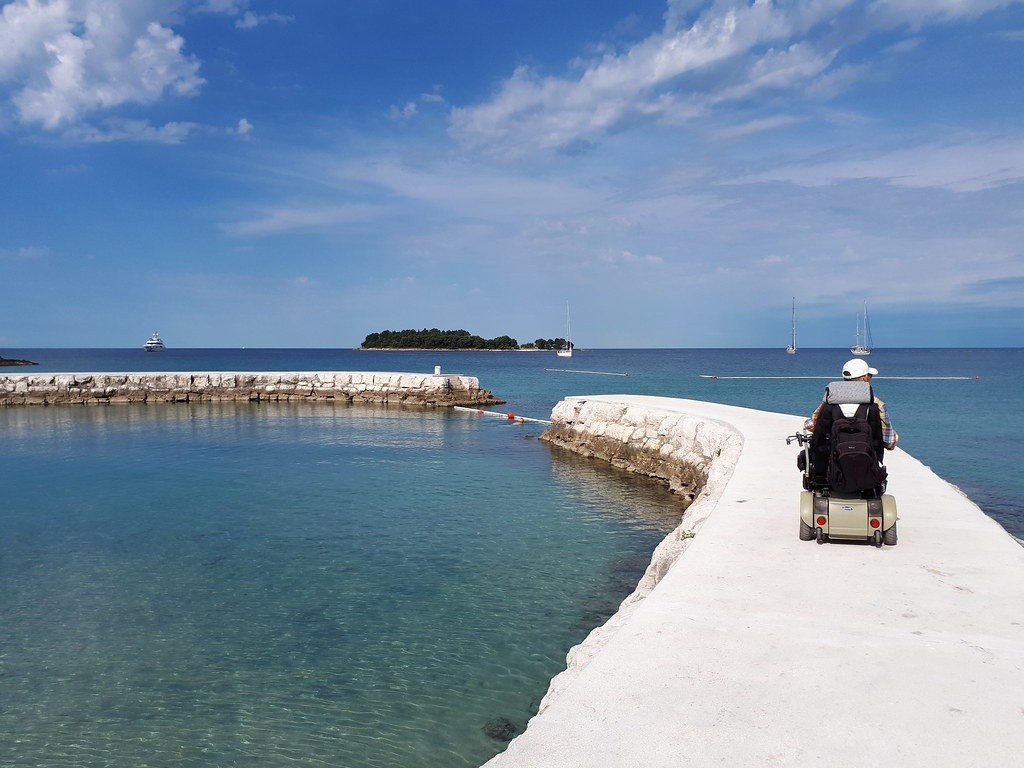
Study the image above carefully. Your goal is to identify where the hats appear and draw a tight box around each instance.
[842,358,878,379]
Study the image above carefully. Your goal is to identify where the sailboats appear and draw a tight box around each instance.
[850,299,874,356]
[557,300,572,357]
[786,296,800,354]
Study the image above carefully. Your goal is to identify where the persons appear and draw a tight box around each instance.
[797,358,900,491]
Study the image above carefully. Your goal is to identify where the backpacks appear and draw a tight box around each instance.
[827,402,883,494]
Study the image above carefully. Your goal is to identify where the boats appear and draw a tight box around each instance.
[144,330,167,352]
